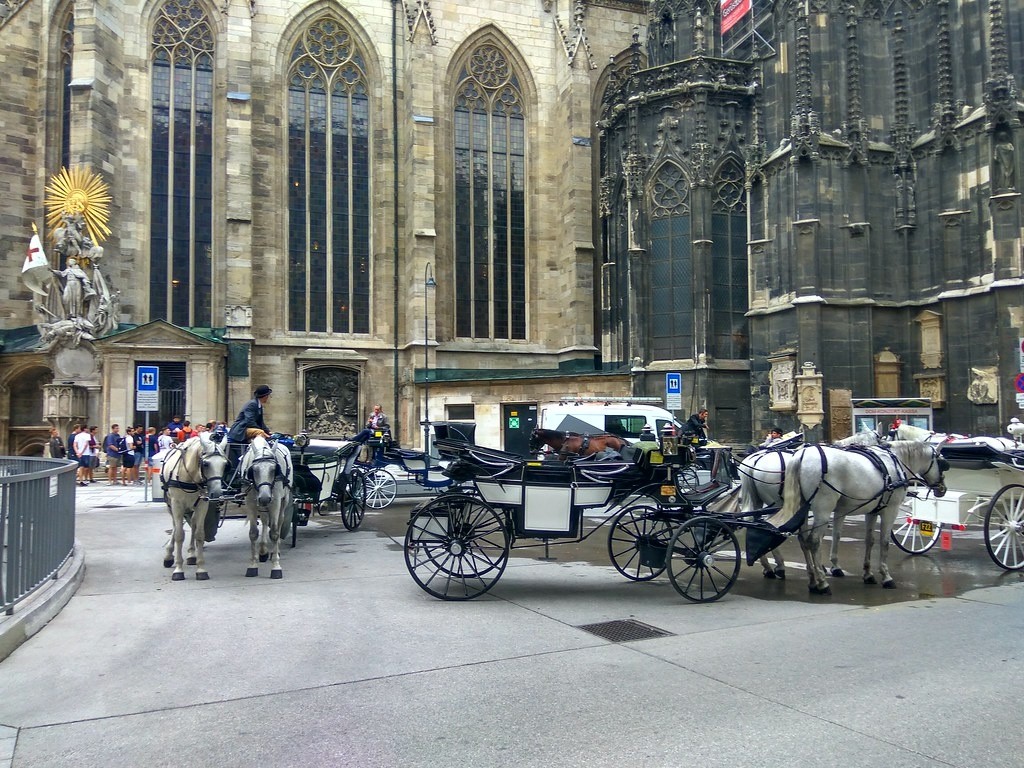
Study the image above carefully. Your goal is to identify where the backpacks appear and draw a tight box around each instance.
[102,434,115,453]
[115,437,132,454]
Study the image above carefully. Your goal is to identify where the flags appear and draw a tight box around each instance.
[20,235,49,297]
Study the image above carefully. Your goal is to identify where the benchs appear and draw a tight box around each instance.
[928,440,1024,473]
[569,446,641,480]
[289,444,337,463]
[400,448,427,470]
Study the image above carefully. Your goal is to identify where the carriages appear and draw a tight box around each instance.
[161,426,403,581]
[529,426,732,497]
[403,428,947,601]
[888,436,1024,570]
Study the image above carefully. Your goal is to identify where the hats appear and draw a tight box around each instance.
[254,385,272,398]
[172,415,182,419]
[772,428,783,434]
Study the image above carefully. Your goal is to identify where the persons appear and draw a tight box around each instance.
[764,428,784,448]
[49,428,67,458]
[147,427,158,485]
[106,424,120,484]
[68,424,81,484]
[73,425,91,485]
[679,409,710,440]
[55,207,83,254]
[166,414,183,437]
[229,385,272,441]
[178,421,197,443]
[49,258,90,318]
[89,426,101,483]
[158,426,174,451]
[343,404,390,443]
[122,424,144,485]
[191,418,228,437]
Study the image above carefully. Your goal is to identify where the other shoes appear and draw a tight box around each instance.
[133,481,144,485]
[90,479,97,483]
[122,484,127,486]
[147,479,153,486]
[80,482,87,486]
[76,481,80,485]
[113,482,122,485]
[106,482,113,486]
[83,481,90,484]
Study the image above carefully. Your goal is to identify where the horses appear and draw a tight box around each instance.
[159,431,228,580]
[241,435,294,579]
[529,424,630,462]
[733,419,1023,596]
